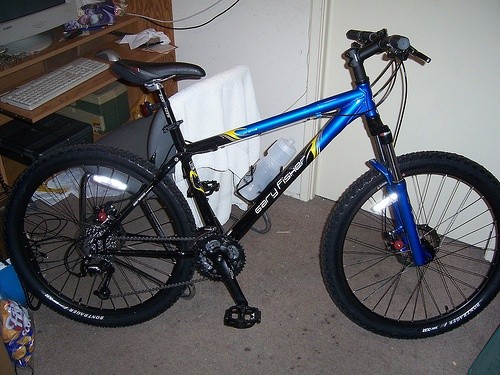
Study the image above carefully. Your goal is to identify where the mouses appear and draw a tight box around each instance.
[96,47,121,62]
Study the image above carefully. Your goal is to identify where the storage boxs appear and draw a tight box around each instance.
[0,258,27,306]
[55,81,131,134]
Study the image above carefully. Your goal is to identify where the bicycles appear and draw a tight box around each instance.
[6,28,500,340]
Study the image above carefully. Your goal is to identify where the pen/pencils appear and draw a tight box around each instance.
[59,23,113,42]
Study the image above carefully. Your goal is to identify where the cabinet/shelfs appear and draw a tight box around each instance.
[1,1,178,185]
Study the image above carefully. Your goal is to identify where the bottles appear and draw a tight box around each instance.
[237,138,296,201]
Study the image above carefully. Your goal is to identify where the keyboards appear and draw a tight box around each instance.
[0,56,111,111]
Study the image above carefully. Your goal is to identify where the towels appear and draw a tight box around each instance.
[168,65,260,230]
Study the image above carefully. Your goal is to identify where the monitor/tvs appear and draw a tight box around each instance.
[0,0,79,55]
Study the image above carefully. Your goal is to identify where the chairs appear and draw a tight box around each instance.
[81,65,271,300]
